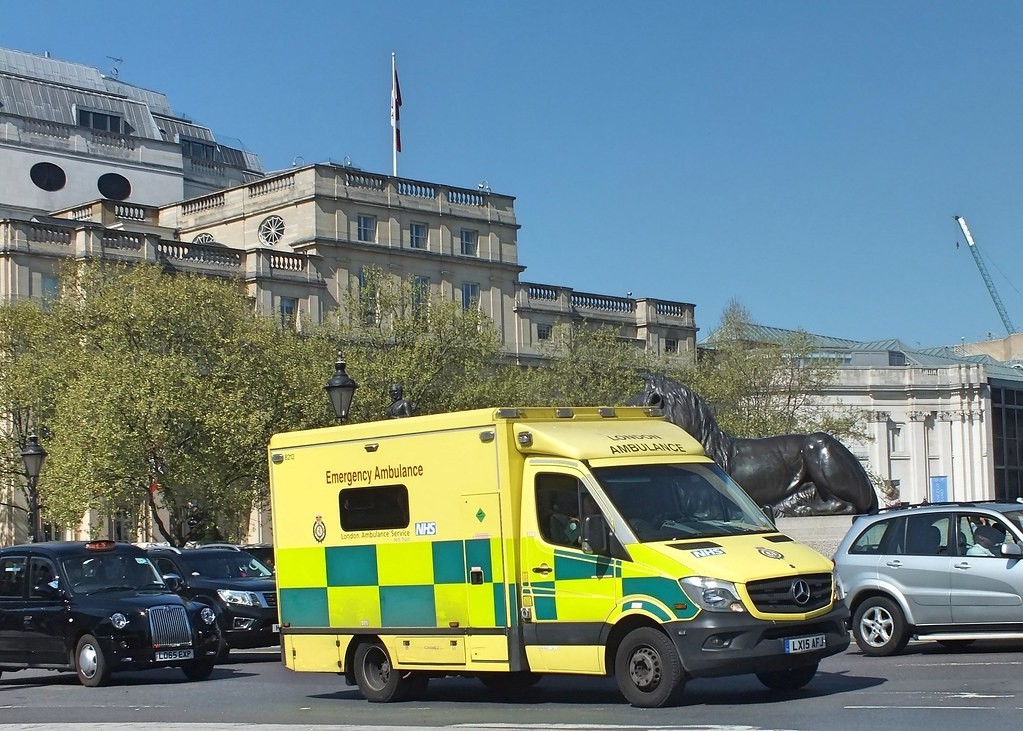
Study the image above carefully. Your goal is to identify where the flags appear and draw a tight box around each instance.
[393,69,406,152]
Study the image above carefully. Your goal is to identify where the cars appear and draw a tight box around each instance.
[0,540,221,687]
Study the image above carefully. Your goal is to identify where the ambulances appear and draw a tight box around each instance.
[262,404,854,709]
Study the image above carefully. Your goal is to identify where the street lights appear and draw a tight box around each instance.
[21,433,48,544]
[324,347,358,425]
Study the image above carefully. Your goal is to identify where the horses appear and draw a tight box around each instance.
[626,372,879,517]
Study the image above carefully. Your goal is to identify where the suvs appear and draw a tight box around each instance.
[144,542,280,649]
[830,497,1023,657]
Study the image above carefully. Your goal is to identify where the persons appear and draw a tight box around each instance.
[566,493,601,548]
[966,523,1006,556]
